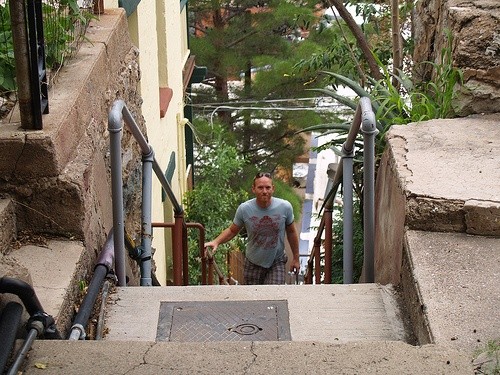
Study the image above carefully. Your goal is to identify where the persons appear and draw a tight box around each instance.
[204,172,300,285]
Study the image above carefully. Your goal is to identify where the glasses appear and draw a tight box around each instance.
[254,173,272,181]
